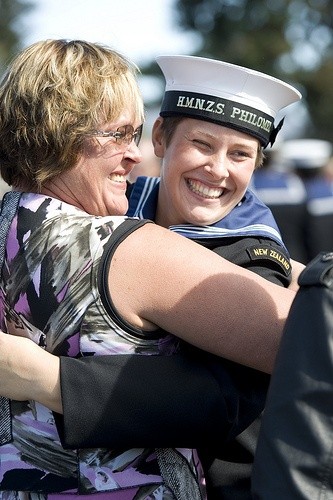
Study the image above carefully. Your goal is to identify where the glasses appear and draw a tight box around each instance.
[92,123,143,148]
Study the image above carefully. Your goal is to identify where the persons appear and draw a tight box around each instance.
[248,131,333,500]
[0,39,310,500]
[0,53,292,500]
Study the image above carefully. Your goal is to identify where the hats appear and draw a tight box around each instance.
[281,139,333,169]
[156,55,303,150]
